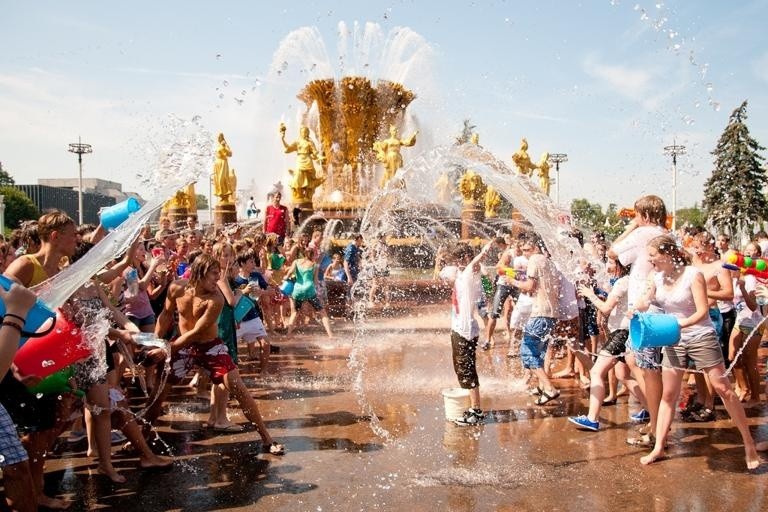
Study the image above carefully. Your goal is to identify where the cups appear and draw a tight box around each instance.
[248,277,261,298]
[151,247,164,259]
[754,284,767,305]
[132,332,157,346]
[124,276,140,298]
[576,272,589,293]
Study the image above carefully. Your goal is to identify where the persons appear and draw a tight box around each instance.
[280,124,327,205]
[213,133,238,200]
[459,132,486,200]
[536,152,552,196]
[373,124,418,190]
[513,138,538,178]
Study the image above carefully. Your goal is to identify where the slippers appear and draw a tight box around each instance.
[528,383,562,407]
[261,441,286,457]
[602,397,619,406]
[204,419,246,434]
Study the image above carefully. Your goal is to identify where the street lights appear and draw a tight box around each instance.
[67,134,93,226]
[546,152,570,203]
[661,137,687,229]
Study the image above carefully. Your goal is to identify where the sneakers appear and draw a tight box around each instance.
[567,414,601,434]
[453,406,486,428]
[626,408,652,422]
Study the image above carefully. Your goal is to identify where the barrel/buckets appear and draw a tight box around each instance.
[99,198,142,231]
[12,306,97,385]
[271,254,286,270]
[234,295,256,323]
[25,364,86,399]
[442,387,473,423]
[629,310,681,351]
[279,279,295,297]
[0,274,57,350]
[177,262,189,276]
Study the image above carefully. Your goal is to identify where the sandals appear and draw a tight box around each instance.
[677,399,718,424]
[624,425,659,449]
